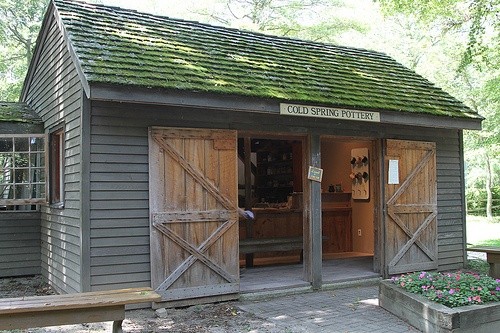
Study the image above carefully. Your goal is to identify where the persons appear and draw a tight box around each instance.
[238,207,248,220]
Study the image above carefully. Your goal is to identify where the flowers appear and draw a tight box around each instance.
[392,271,500,307]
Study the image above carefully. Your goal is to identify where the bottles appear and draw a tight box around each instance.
[252,150,294,208]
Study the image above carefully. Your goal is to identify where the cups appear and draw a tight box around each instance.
[328,183,335,192]
[335,184,341,192]
[349,157,368,180]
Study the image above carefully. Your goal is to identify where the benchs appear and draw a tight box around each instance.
[239,235,329,267]
[0,286,161,333]
